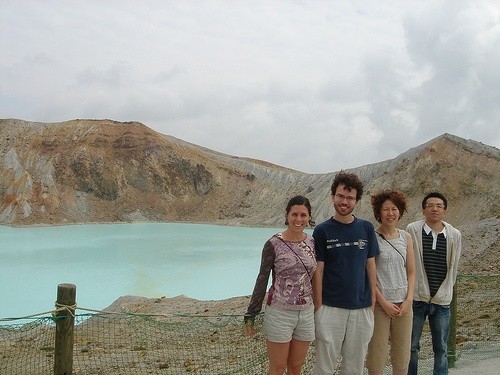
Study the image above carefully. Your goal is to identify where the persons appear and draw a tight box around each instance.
[312,173,380,375]
[244,195,318,375]
[367,188,415,375]
[406,193,461,375]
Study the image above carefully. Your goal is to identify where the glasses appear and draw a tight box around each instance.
[424,203,445,209]
[334,193,356,201]
